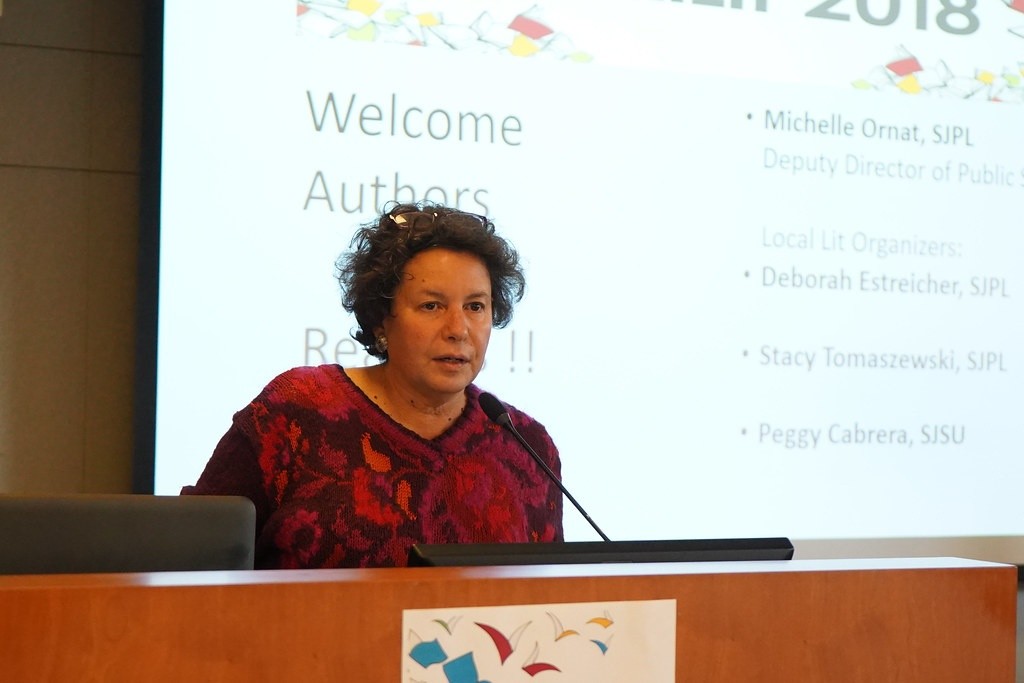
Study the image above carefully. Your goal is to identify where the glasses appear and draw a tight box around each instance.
[389,210,487,231]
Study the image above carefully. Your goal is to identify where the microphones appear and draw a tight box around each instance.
[478,392,610,543]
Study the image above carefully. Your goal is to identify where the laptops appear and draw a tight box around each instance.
[0,493,258,574]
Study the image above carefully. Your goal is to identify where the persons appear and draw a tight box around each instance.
[181,205,565,570]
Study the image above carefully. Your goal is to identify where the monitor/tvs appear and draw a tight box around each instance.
[403,538,794,566]
